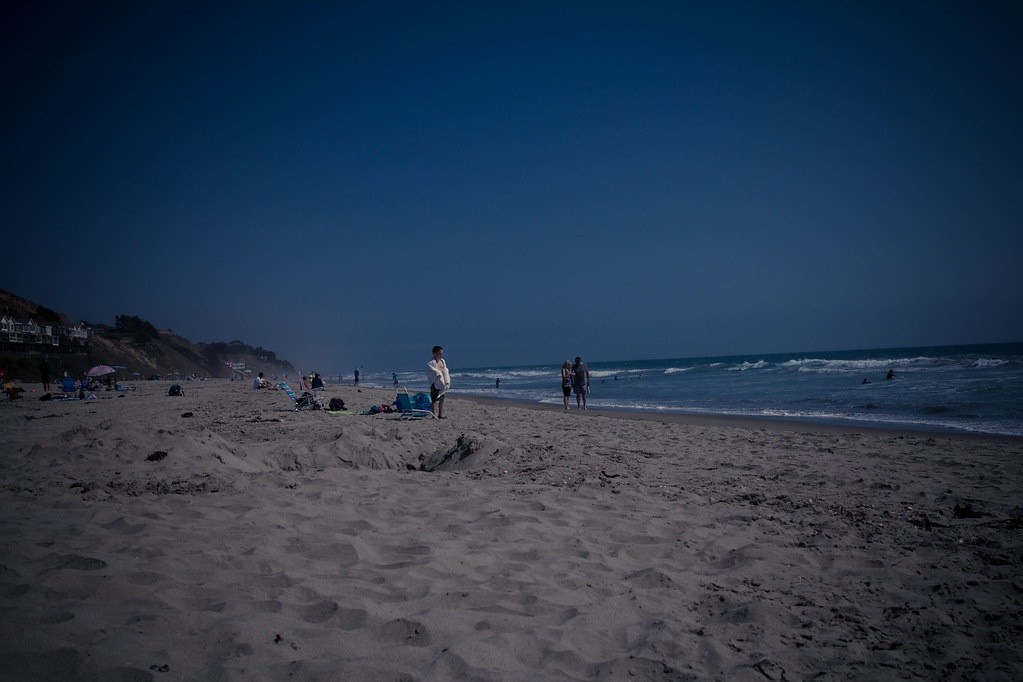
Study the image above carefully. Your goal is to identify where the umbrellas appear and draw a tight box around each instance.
[82,359,253,384]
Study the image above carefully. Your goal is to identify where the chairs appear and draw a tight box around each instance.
[394,386,439,421]
[279,381,317,412]
[61,377,81,400]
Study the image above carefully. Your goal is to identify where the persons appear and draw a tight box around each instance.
[495,377,501,389]
[392,372,400,387]
[598,368,649,388]
[859,364,902,386]
[298,367,329,397]
[561,359,575,410]
[253,372,273,389]
[354,365,360,387]
[426,345,451,422]
[571,356,591,412]
[0,363,135,411]
